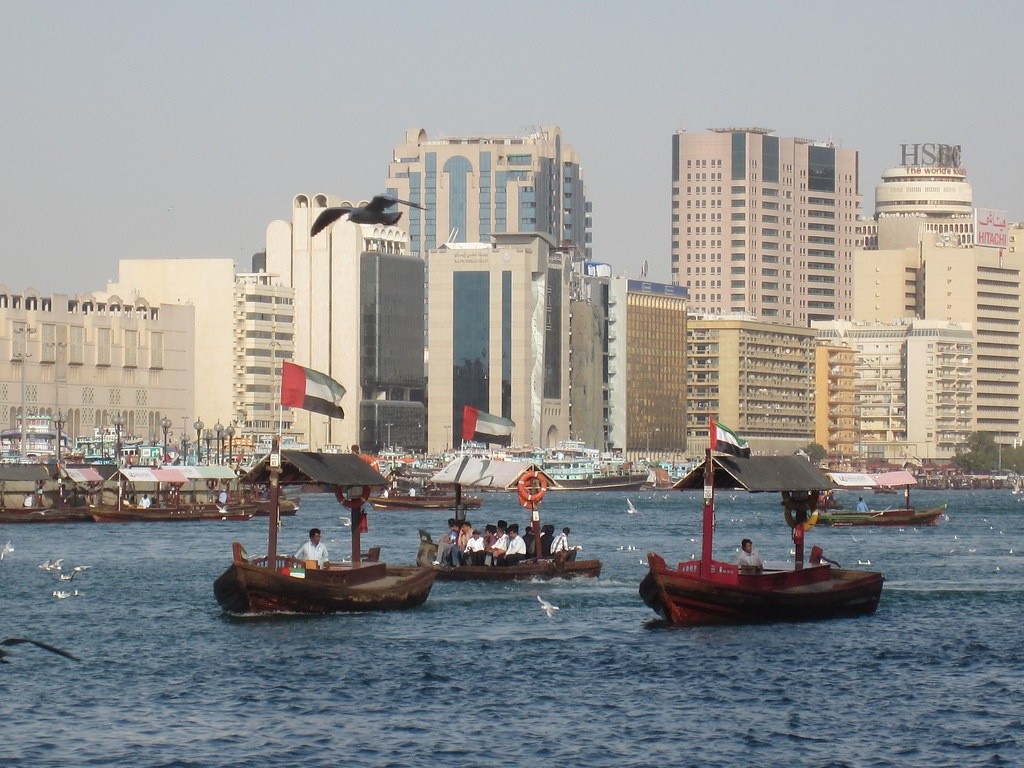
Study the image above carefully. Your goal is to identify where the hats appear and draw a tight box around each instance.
[222,488,226,492]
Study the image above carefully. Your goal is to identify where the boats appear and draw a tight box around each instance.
[368,463,481,512]
[411,508,599,581]
[210,437,439,612]
[0,464,87,521]
[88,463,257,521]
[551,473,651,491]
[873,488,897,494]
[640,447,884,622]
[805,498,947,525]
[252,486,300,514]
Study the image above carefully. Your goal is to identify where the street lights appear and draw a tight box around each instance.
[220,428,231,465]
[214,422,223,464]
[112,410,126,463]
[193,418,204,462]
[52,408,68,464]
[160,415,172,465]
[181,433,190,462]
[226,425,236,466]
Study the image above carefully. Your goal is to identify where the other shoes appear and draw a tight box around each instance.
[432,561,440,566]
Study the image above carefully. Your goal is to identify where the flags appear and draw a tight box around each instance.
[709,415,750,458]
[462,406,514,445]
[281,361,346,419]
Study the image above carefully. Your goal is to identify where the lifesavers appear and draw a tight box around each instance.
[358,453,380,473]
[57,460,67,469]
[520,481,543,508]
[206,479,217,489]
[518,472,547,501]
[336,484,370,507]
[785,496,818,532]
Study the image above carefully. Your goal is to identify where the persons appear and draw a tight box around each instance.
[735,538,763,573]
[432,518,580,566]
[857,497,868,512]
[292,528,328,570]
[23,480,269,508]
